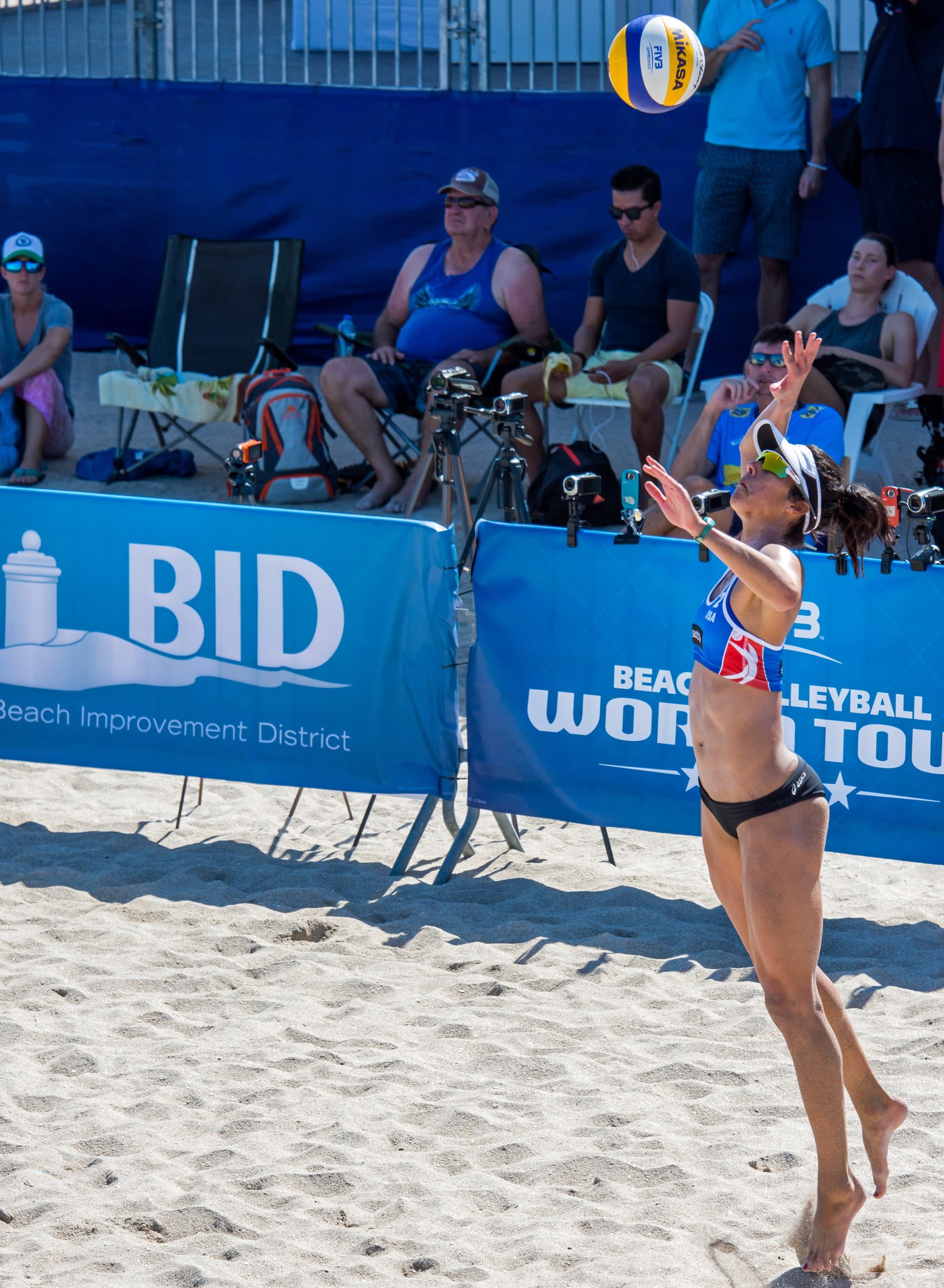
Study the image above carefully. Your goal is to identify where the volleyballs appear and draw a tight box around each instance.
[607,14,705,114]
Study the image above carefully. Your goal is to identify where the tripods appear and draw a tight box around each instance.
[478,435,532,525]
[405,425,478,562]
[225,458,258,506]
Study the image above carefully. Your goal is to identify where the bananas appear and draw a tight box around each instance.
[542,351,572,400]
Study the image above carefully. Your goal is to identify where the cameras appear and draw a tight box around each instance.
[691,490,731,515]
[491,391,529,419]
[430,365,483,396]
[907,487,944,517]
[562,472,602,497]
[228,439,264,464]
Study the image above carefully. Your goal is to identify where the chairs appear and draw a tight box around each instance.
[315,243,574,505]
[98,233,305,486]
[701,267,939,489]
[544,291,715,477]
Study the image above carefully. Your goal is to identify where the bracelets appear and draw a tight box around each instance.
[569,352,587,368]
[806,161,828,171]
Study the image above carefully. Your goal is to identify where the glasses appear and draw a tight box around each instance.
[609,204,651,220]
[4,259,43,273]
[749,352,786,367]
[444,196,492,209]
[755,449,816,520]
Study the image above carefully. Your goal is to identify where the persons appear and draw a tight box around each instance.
[0,230,76,487]
[491,165,701,512]
[319,161,547,514]
[859,0,944,394]
[786,230,919,450]
[621,322,846,553]
[643,330,910,1275]
[692,0,836,334]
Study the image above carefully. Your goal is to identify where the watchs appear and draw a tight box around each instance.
[693,517,715,545]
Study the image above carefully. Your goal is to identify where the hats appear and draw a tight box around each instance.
[1,232,44,264]
[753,419,822,535]
[438,167,499,205]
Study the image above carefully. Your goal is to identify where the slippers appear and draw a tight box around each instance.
[8,467,45,486]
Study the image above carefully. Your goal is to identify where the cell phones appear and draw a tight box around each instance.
[880,486,901,527]
[622,470,641,509]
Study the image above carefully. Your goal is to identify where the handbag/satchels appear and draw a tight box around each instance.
[76,446,197,482]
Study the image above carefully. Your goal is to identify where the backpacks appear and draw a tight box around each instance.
[525,440,624,530]
[239,369,339,503]
